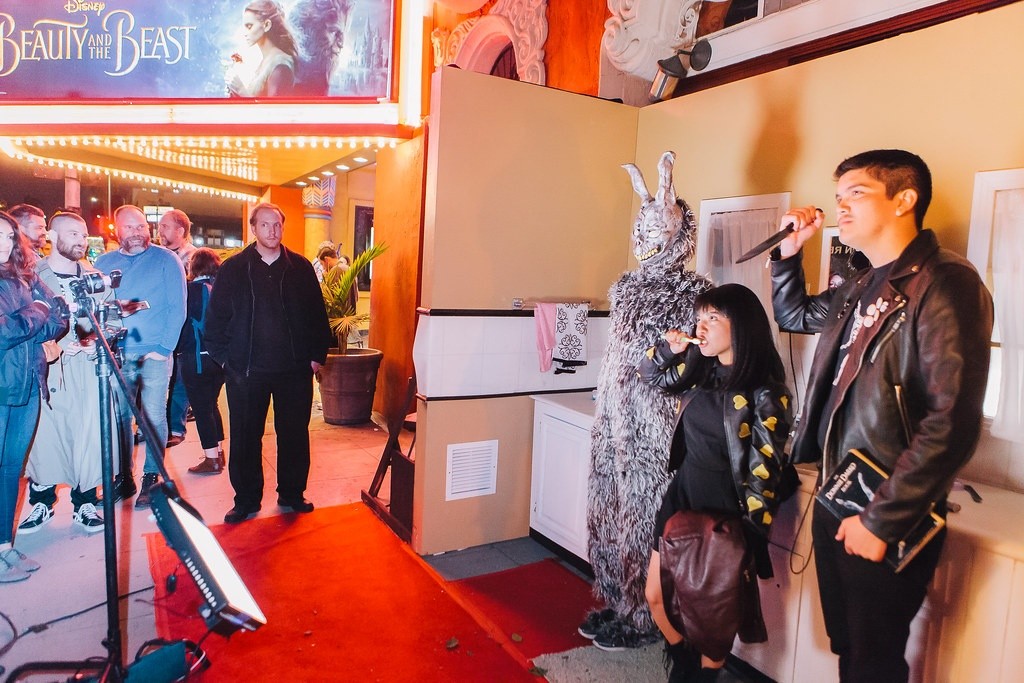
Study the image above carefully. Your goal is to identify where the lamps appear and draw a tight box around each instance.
[648,40,711,103]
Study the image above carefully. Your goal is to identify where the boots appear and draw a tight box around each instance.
[663,632,722,683]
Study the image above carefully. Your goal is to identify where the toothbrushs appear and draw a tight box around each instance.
[660,334,702,345]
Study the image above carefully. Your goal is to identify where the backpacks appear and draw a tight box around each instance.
[188,280,213,337]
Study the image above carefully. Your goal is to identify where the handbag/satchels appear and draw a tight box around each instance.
[42,339,63,362]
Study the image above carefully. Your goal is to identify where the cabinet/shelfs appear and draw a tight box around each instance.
[730,475,1024,683]
[525,394,605,561]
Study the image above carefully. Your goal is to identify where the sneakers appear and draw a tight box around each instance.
[18,502,105,533]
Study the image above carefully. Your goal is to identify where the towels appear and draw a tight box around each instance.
[532,301,589,375]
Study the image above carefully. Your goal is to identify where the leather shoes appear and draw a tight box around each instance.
[95,473,159,510]
[224,493,313,523]
[0,548,40,583]
[188,449,225,474]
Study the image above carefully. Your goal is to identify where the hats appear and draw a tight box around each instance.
[319,241,336,250]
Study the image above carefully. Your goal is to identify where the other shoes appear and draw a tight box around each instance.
[134,433,146,445]
[167,436,185,448]
[186,407,196,422]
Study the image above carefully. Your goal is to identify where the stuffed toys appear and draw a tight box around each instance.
[578,150,718,651]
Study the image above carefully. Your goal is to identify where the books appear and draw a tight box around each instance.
[814,449,945,572]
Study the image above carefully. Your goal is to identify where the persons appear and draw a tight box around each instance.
[764,150,995,683]
[313,241,359,410]
[637,284,793,683]
[0,204,123,582]
[93,205,226,511]
[203,203,330,524]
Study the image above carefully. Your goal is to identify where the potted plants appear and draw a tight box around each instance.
[318,242,387,426]
